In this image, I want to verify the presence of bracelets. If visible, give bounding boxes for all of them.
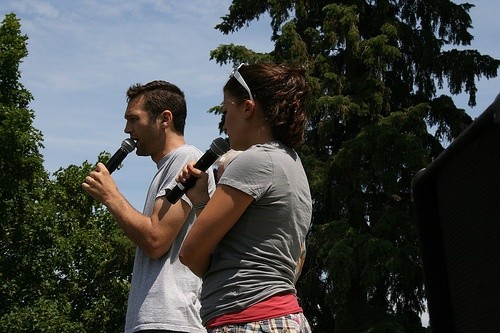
[191,199,209,210]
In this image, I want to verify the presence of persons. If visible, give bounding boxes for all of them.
[82,79,216,333]
[175,60,317,333]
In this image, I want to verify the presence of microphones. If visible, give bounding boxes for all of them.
[166,137,229,204]
[105,138,135,175]
